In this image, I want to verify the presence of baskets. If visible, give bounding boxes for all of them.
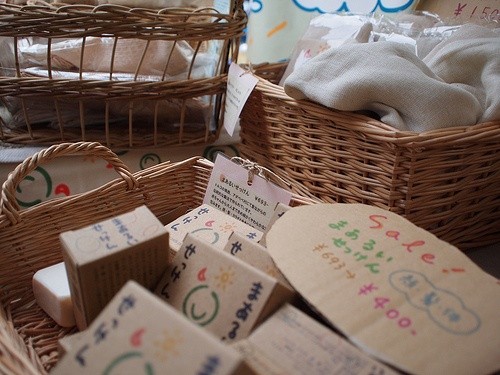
[0,141,324,375]
[239,60,500,251]
[0,0,248,149]
